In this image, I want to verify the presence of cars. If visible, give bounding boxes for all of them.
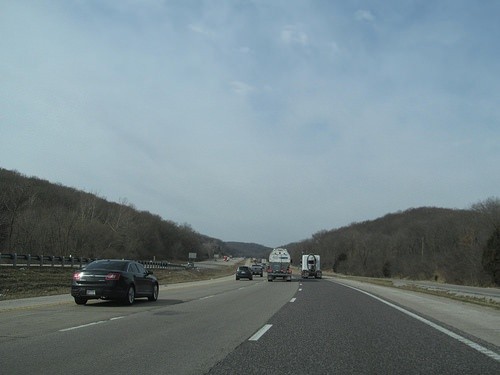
[187,260,194,267]
[251,264,264,277]
[235,265,253,281]
[71,260,159,305]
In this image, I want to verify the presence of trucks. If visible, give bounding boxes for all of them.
[301,253,323,279]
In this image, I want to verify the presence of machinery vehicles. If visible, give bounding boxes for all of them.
[266,247,293,282]
